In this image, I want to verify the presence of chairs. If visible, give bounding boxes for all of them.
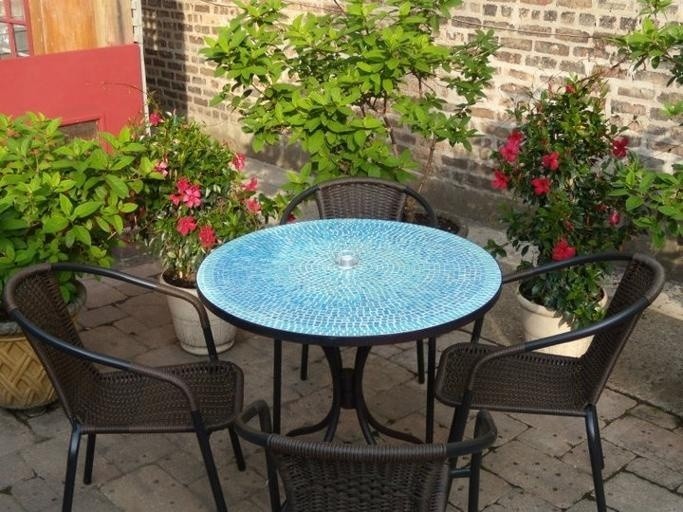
[435,251,667,512]
[235,398,497,512]
[279,178,436,384]
[4,261,244,512]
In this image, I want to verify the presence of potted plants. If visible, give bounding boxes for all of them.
[0,111,164,411]
[199,0,505,241]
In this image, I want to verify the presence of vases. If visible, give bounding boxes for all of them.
[159,272,236,356]
[514,284,608,356]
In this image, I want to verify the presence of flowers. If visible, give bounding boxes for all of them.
[480,75,642,330]
[121,112,295,282]
